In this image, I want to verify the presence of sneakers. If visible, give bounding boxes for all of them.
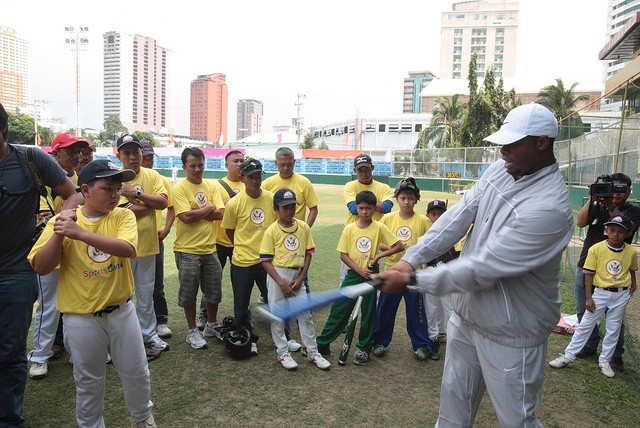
[185,326,207,349]
[307,353,331,370]
[598,361,615,378]
[29,362,48,380]
[373,340,385,356]
[301,346,331,356]
[575,350,596,357]
[437,333,448,344]
[353,348,368,366]
[202,323,223,340]
[257,296,264,304]
[148,336,170,351]
[414,347,427,360]
[272,339,302,353]
[549,352,571,369]
[277,353,298,370]
[249,342,258,355]
[69,353,111,365]
[195,321,206,330]
[609,357,624,372]
[155,324,172,338]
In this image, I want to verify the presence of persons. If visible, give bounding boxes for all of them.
[66,137,96,365]
[29,135,88,380]
[0,103,77,426]
[260,147,318,352]
[342,155,394,321]
[113,134,169,352]
[259,189,330,371]
[425,201,439,361]
[574,173,639,373]
[372,177,430,360]
[370,101,574,428]
[196,150,256,331]
[138,139,175,337]
[221,159,291,347]
[27,158,155,428]
[549,215,638,378]
[171,147,224,348]
[301,191,405,365]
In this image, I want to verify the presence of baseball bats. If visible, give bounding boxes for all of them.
[338,263,373,366]
[254,279,384,323]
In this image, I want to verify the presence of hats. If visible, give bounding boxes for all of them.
[140,141,158,158]
[74,159,137,192]
[79,137,96,152]
[482,102,559,146]
[117,134,143,153]
[603,215,631,232]
[426,200,446,214]
[273,188,300,207]
[239,157,267,177]
[50,131,89,151]
[354,154,372,171]
[392,177,421,201]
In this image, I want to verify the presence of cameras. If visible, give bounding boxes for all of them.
[589,174,628,203]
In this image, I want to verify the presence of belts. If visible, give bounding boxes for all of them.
[94,298,131,318]
[597,287,627,292]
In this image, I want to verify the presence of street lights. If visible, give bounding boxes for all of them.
[64,27,89,137]
